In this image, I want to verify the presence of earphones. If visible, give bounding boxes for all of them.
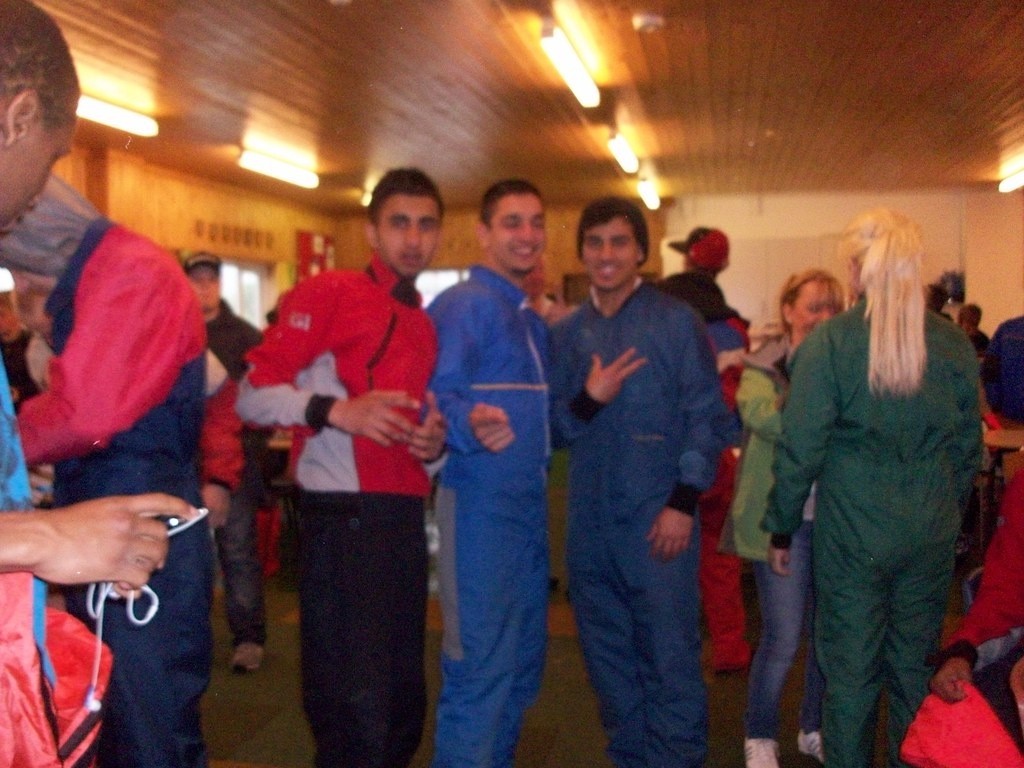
[85,685,101,713]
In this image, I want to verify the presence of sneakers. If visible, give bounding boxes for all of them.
[797,729,825,764]
[744,737,781,768]
[231,642,264,671]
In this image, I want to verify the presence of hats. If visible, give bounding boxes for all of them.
[183,251,222,273]
[670,226,729,270]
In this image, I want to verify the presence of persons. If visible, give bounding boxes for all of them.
[666,204,1023,767]
[552,197,723,767]
[236,165,450,768]
[0,216,263,768]
[421,177,550,768]
[0,0,200,768]
